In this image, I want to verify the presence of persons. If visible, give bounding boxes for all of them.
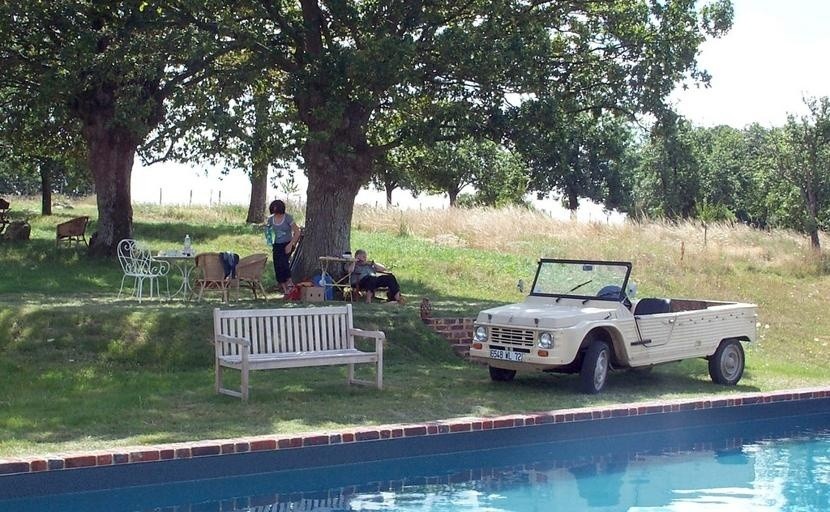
[347,249,400,304]
[265,200,300,293]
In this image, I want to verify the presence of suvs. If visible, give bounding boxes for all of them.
[490,448,758,512]
[469,257,759,391]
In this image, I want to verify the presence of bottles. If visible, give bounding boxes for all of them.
[266,230,273,246]
[184,234,191,254]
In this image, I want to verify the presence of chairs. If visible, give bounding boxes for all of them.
[634,297,673,315]
[356,260,388,301]
[117,238,170,304]
[596,286,632,310]
[188,251,239,303]
[55,217,89,249]
[228,253,269,303]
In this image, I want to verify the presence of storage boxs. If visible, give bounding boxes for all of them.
[299,287,324,304]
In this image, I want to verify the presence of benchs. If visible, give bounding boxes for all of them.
[213,303,387,404]
